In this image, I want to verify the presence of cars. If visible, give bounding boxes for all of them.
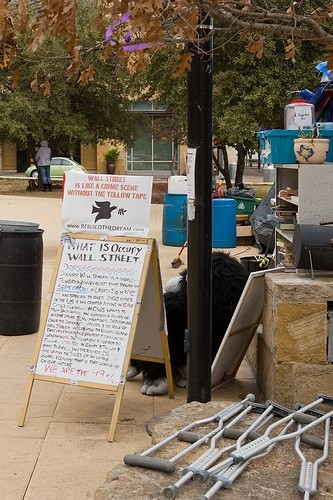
[25,156,88,184]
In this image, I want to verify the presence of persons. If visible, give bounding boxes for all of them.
[34,140,53,192]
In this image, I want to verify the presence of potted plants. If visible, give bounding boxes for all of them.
[293,122,331,163]
[102,147,120,174]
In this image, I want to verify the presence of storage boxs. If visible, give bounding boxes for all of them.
[318,127,333,163]
[258,128,315,163]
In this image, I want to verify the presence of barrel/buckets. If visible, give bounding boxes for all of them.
[0,220,44,336]
[211,197,237,248]
[263,170,275,182]
[162,193,187,247]
[230,165,237,179]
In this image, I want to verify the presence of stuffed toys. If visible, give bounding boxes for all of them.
[124,249,247,397]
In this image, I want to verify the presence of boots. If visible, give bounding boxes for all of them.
[48,184,52,191]
[41,184,49,192]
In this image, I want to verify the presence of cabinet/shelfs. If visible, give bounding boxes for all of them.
[272,159,333,274]
